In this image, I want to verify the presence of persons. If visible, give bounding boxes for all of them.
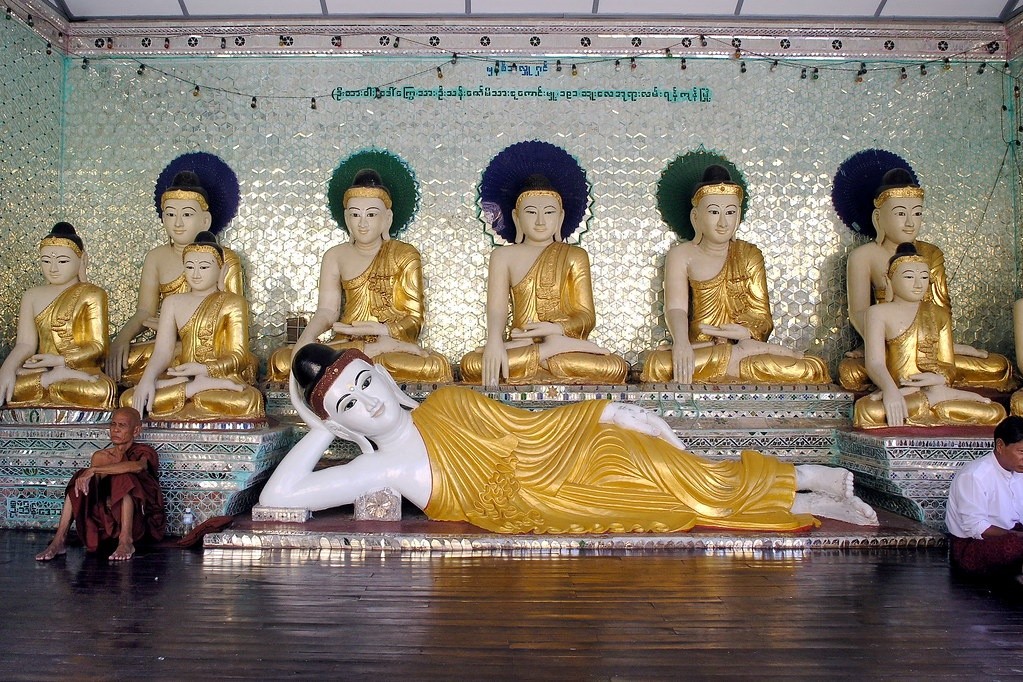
[851,242,1007,425]
[100,170,260,389]
[35,406,173,560]
[257,342,880,536]
[458,174,629,385]
[946,415,1023,604]
[268,169,453,383]
[839,167,1011,389]
[119,231,267,419]
[640,166,832,384]
[0,221,117,410]
